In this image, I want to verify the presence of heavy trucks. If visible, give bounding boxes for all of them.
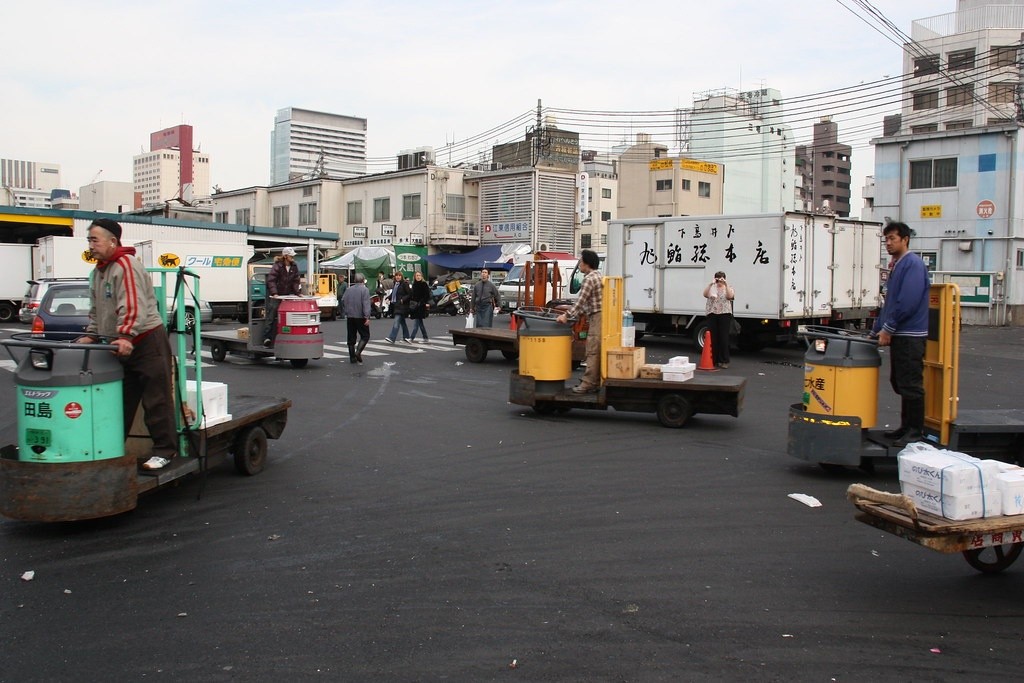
[549,210,884,355]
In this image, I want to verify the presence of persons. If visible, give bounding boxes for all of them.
[341,273,371,365]
[868,224,930,448]
[75,219,177,469]
[557,249,604,393]
[385,271,409,344]
[337,275,348,320]
[470,268,503,327]
[405,271,430,344]
[263,247,303,345]
[375,271,410,292]
[703,271,735,369]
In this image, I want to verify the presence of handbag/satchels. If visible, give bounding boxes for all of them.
[466,311,474,329]
[408,300,417,309]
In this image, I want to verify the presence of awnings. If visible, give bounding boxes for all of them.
[537,252,577,260]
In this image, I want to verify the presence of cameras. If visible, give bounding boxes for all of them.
[716,280,722,283]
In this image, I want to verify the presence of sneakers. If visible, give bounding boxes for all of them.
[143,454,171,468]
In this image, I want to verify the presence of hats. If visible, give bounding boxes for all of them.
[282,246,298,257]
[92,217,122,246]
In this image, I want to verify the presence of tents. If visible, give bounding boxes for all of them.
[318,246,396,287]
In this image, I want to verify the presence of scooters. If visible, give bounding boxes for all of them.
[380,280,475,319]
[364,279,385,319]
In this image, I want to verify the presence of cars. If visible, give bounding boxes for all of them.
[29,283,91,341]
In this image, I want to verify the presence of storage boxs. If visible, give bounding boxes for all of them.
[185,380,232,430]
[899,451,1024,520]
[237,326,249,340]
[607,314,697,382]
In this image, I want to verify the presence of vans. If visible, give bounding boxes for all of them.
[498,259,577,314]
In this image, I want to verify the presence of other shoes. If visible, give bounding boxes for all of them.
[400,339,407,344]
[893,427,922,447]
[884,426,909,439]
[406,338,413,346]
[386,337,395,344]
[419,339,431,344]
[263,338,271,346]
[572,378,596,394]
[722,361,730,369]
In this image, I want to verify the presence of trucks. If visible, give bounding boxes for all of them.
[136,239,273,324]
[0,235,141,324]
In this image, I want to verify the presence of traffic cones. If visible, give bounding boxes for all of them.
[696,330,720,371]
[509,314,518,331]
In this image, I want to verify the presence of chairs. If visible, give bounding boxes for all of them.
[57,303,76,314]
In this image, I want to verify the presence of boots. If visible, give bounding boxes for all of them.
[349,346,357,364]
[355,340,367,363]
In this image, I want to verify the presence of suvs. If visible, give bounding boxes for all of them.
[18,277,214,334]
